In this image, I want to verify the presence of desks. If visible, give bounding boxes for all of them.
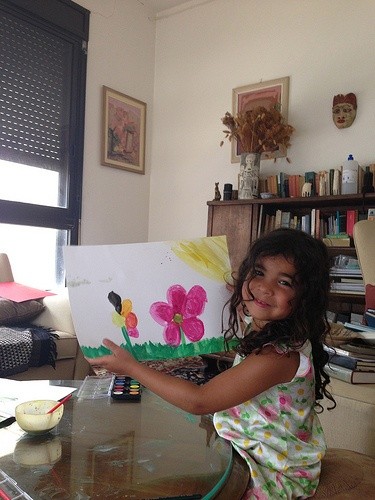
[0,377,249,500]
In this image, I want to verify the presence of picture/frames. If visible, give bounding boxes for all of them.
[232,75,290,163]
[101,84,147,174]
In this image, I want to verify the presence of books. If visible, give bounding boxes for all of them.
[264,209,358,247]
[323,344,375,385]
[259,170,341,199]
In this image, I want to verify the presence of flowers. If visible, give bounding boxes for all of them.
[220,101,296,166]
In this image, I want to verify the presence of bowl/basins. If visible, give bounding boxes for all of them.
[260,193,275,198]
[14,399,64,436]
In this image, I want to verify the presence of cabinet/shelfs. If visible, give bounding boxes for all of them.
[206,193,375,300]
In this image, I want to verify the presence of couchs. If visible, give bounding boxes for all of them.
[0,252,89,380]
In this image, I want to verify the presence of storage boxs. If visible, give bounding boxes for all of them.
[323,237,351,248]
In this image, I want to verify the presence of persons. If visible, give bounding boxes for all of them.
[83,228,336,500]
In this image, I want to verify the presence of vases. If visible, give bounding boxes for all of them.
[237,151,261,199]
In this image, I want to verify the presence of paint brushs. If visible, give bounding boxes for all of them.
[46,392,73,414]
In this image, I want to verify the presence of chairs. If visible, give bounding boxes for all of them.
[352,220,375,312]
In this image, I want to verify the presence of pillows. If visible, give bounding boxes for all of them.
[307,448,375,499]
[0,298,45,326]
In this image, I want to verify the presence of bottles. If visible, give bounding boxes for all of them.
[341,154,358,195]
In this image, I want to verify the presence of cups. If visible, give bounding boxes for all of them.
[223,184,233,200]
